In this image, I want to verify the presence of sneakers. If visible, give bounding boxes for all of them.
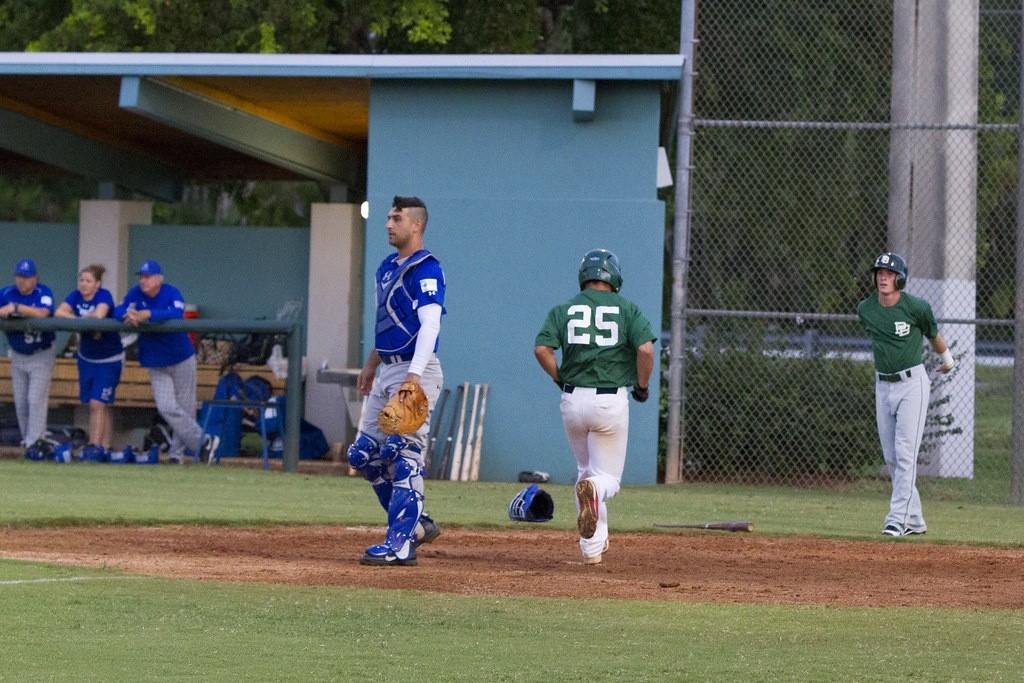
[360,543,418,566]
[903,527,927,537]
[881,523,902,537]
[168,457,184,466]
[583,538,610,564]
[575,479,599,539]
[197,434,220,465]
[411,512,441,550]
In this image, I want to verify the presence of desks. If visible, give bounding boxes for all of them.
[196,400,286,469]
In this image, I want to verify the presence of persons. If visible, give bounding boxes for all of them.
[110,260,220,465]
[346,195,447,567]
[857,254,956,536]
[533,249,658,564]
[55,263,125,463]
[0,258,56,460]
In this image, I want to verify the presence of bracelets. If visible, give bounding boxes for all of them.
[14,302,19,311]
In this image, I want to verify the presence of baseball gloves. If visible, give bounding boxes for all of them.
[377,380,429,436]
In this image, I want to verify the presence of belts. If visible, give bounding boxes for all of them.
[563,385,619,395]
[380,353,413,365]
[877,370,911,384]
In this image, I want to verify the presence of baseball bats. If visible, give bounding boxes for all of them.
[421,381,489,481]
[653,521,755,533]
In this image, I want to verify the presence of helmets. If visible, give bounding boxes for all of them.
[578,249,623,292]
[135,260,161,276]
[14,258,36,278]
[508,482,554,522]
[870,252,908,289]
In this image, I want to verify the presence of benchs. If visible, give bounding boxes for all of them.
[1,358,288,409]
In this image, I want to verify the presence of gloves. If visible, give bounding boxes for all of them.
[631,385,650,403]
[935,349,955,375]
